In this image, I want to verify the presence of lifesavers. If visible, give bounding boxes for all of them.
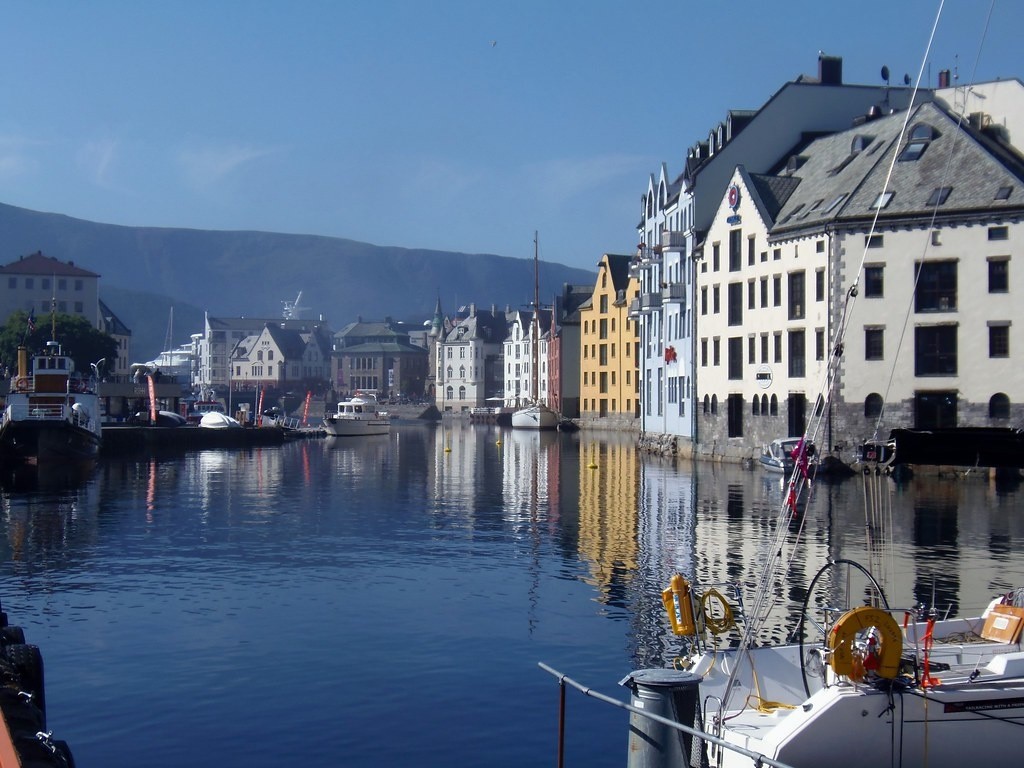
[828,605,905,680]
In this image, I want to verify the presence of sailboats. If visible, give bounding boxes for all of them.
[621,17,1024,768]
[510,229,561,430]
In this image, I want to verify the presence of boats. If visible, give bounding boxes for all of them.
[187,384,226,426]
[200,411,241,429]
[758,438,827,475]
[133,411,187,427]
[322,394,390,436]
[0,273,103,480]
